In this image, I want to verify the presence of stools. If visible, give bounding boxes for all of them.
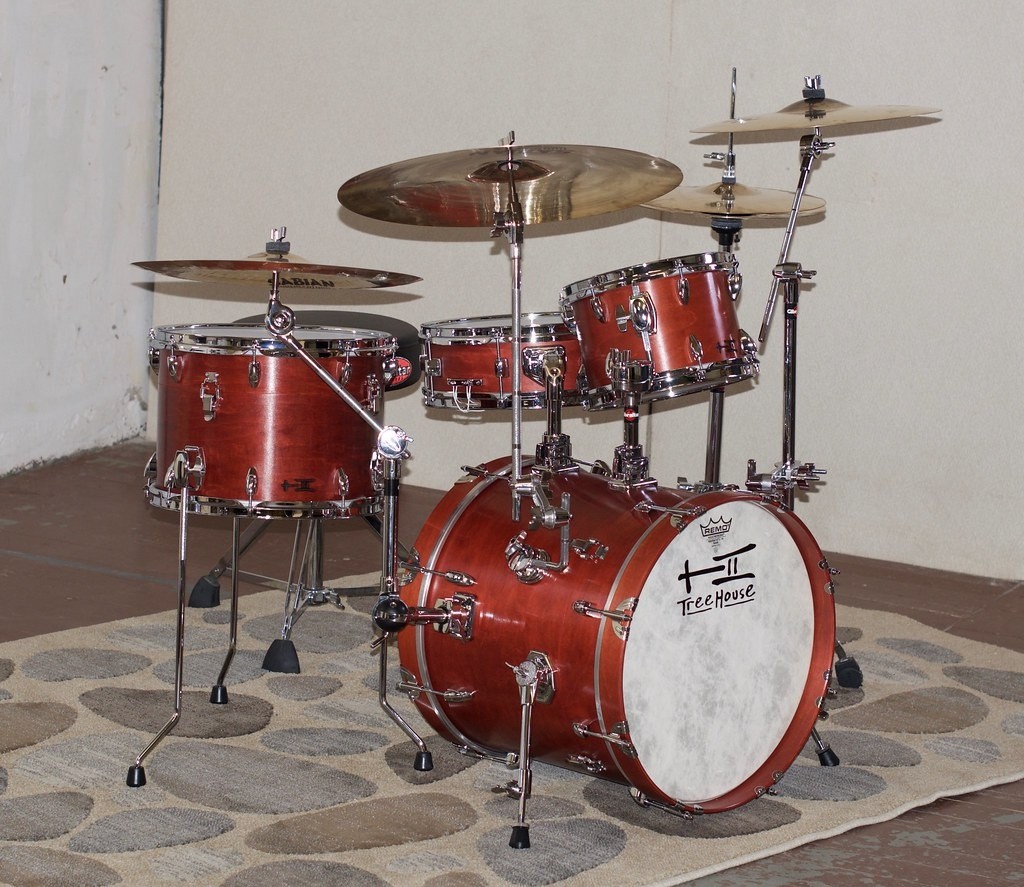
[184,308,421,679]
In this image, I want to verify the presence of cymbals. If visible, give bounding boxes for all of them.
[637,179,827,217]
[336,142,684,227]
[689,97,944,134]
[128,259,424,290]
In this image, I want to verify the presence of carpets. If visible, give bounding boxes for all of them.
[1,566,1024,886]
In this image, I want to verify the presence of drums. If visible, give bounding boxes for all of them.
[418,312,592,410]
[561,252,760,410]
[147,324,397,519]
[397,455,835,815]
